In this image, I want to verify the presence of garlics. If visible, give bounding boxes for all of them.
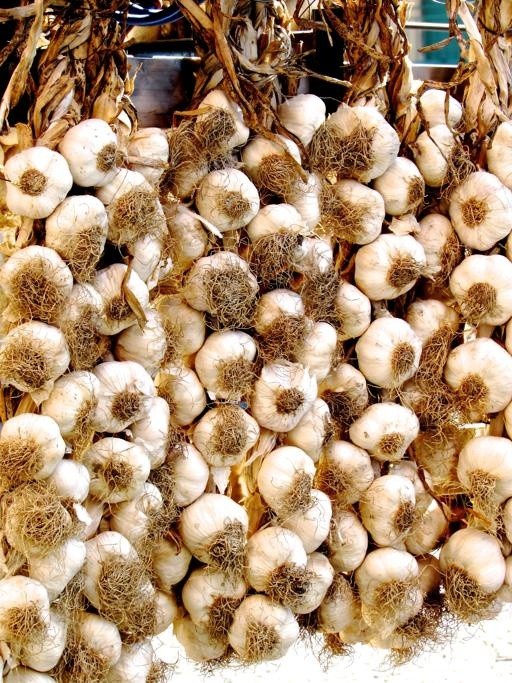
[0,87,511,681]
